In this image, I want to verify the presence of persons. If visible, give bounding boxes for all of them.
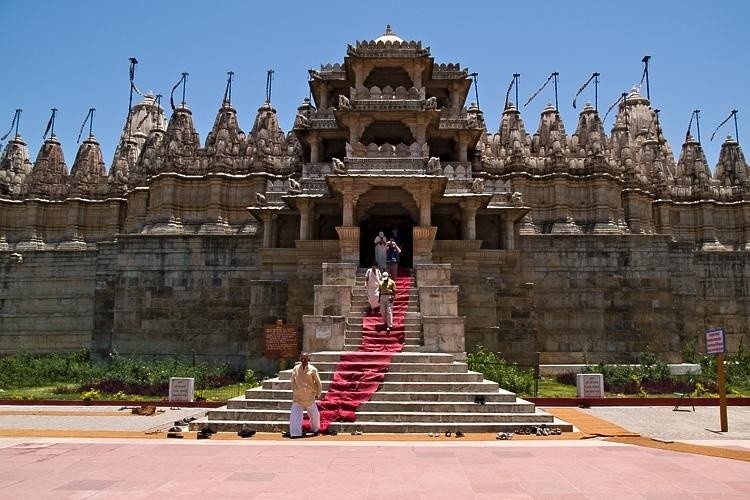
[390,224,400,242]
[377,271,397,330]
[374,231,387,273]
[384,237,402,280]
[364,262,392,315]
[288,352,323,438]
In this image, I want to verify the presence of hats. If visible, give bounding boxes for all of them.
[381,272,389,280]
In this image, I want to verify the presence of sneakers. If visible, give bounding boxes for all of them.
[428,424,561,440]
[167,417,256,439]
